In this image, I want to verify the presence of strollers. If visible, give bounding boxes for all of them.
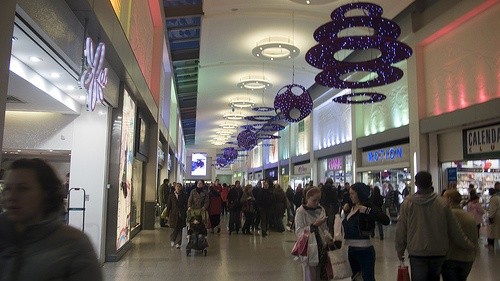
[186,207,208,257]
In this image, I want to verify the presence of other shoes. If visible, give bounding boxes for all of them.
[176,243,181,249]
[171,242,175,247]
[236,231,238,234]
[229,232,231,235]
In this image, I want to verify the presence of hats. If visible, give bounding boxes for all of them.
[415,171,432,187]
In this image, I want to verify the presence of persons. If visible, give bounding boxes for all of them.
[158,170,500,281]
[0,157,104,281]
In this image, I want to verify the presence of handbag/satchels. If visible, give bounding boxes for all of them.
[397,265,410,281]
[290,234,309,255]
[323,240,353,281]
[307,233,319,267]
